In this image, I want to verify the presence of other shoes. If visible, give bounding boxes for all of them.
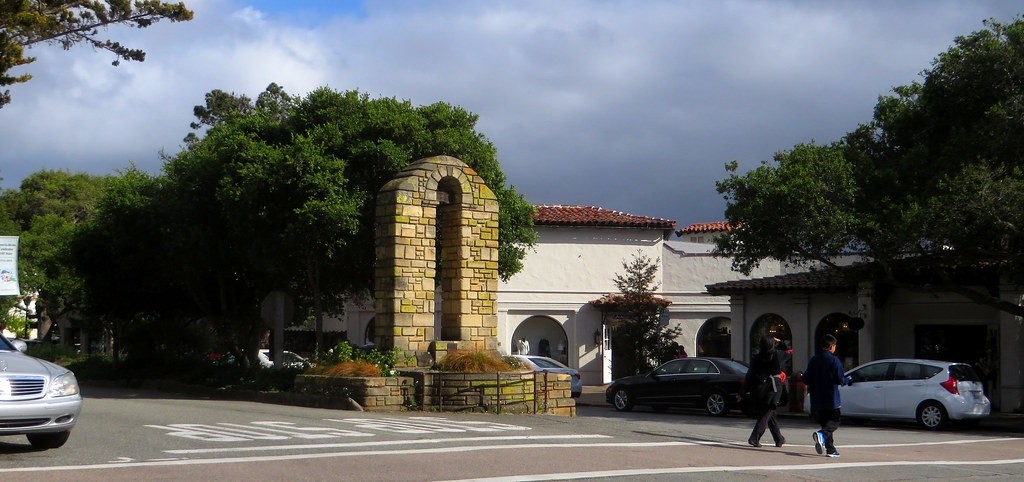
[776,437,786,447]
[749,439,761,447]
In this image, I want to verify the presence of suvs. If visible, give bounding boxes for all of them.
[803,358,993,432]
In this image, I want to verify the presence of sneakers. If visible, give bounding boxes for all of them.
[826,451,840,457]
[813,432,825,454]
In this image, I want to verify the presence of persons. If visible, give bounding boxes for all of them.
[673,346,687,359]
[518,338,530,355]
[743,333,792,447]
[802,334,851,457]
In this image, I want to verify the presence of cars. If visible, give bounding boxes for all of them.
[502,354,583,398]
[0,329,83,452]
[212,348,314,369]
[606,357,790,418]
